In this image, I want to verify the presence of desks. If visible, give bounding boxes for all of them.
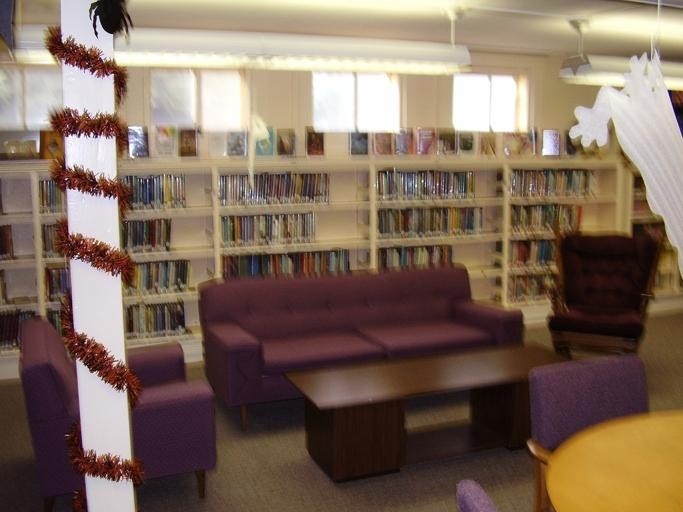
[543,404,683,512]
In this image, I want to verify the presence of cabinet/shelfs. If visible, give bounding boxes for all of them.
[0,160,72,355]
[507,155,683,320]
[120,151,505,356]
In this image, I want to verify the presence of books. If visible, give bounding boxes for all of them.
[121,217,171,254]
[220,173,329,206]
[503,130,584,158]
[509,241,557,268]
[375,168,475,200]
[510,204,582,232]
[221,212,316,247]
[507,274,556,303]
[226,128,324,158]
[222,249,350,280]
[378,244,453,271]
[123,260,191,297]
[124,301,185,340]
[511,169,590,196]
[117,174,187,211]
[349,127,496,157]
[126,125,200,158]
[377,207,483,240]
[0,131,72,350]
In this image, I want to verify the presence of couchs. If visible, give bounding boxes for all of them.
[195,264,522,429]
[544,227,660,357]
[13,312,220,499]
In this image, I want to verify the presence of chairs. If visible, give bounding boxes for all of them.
[524,352,651,512]
[456,478,498,511]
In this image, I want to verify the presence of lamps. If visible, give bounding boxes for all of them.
[560,17,683,93]
[8,0,472,79]
